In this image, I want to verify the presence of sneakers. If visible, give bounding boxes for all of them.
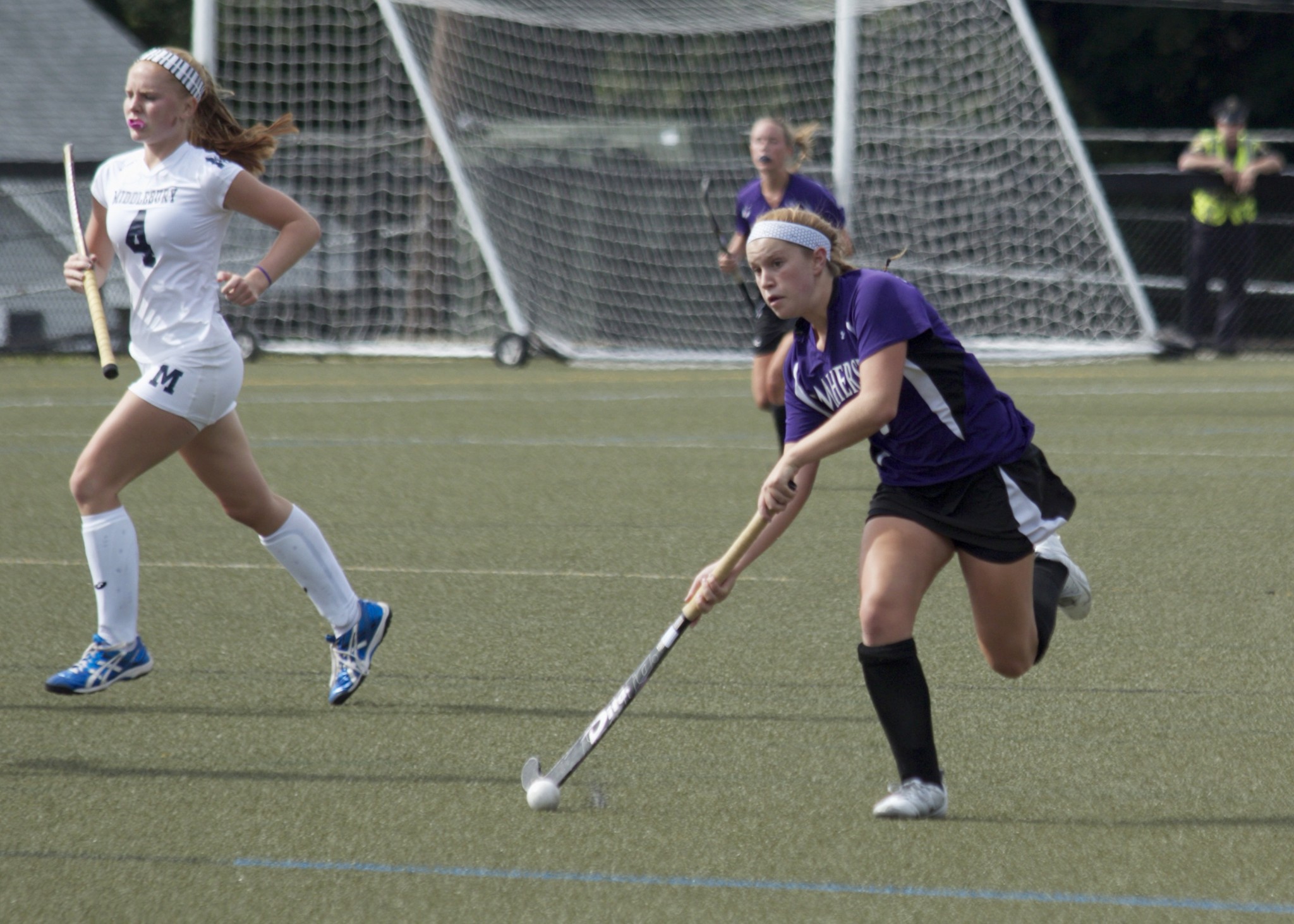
[873,779,948,818]
[326,599,393,706]
[45,634,153,696]
[1033,534,1090,621]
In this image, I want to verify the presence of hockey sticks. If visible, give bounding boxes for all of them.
[701,175,757,318]
[62,142,119,379]
[520,479,797,794]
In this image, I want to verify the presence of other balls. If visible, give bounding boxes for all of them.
[525,778,563,810]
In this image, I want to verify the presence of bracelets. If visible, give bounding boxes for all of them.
[255,266,272,286]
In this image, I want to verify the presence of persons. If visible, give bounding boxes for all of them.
[718,114,845,454]
[45,46,390,708]
[684,207,1094,815]
[1146,95,1286,359]
